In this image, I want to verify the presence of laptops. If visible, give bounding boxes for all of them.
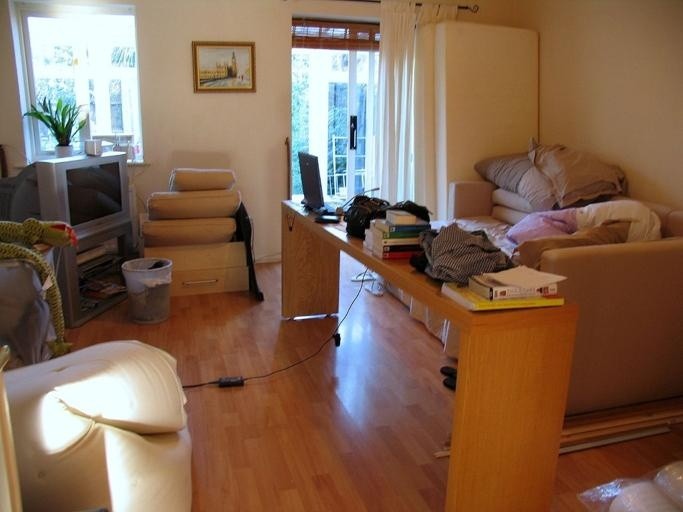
[297,150,352,215]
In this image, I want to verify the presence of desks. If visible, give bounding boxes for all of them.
[281,198,580,512]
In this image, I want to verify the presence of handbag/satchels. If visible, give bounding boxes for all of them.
[344,196,390,240]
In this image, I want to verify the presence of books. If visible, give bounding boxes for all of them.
[469,274,558,301]
[441,281,564,312]
[362,210,431,262]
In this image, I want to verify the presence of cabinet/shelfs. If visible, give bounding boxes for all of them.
[52,222,134,329]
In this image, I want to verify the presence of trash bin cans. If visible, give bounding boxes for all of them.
[121,258,172,324]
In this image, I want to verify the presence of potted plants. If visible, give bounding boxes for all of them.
[23,96,88,157]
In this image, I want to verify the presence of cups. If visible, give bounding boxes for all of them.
[84,139,102,157]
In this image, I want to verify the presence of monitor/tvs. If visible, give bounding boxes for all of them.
[33,151,133,236]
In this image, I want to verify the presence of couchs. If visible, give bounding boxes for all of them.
[448,181,683,416]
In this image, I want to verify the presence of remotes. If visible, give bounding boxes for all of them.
[316,211,342,225]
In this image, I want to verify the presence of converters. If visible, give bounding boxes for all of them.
[215,374,246,386]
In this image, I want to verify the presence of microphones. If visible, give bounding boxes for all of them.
[335,187,380,215]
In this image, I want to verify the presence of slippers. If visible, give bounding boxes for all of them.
[440,367,456,390]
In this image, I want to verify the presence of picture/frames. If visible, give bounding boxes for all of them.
[191,41,256,94]
